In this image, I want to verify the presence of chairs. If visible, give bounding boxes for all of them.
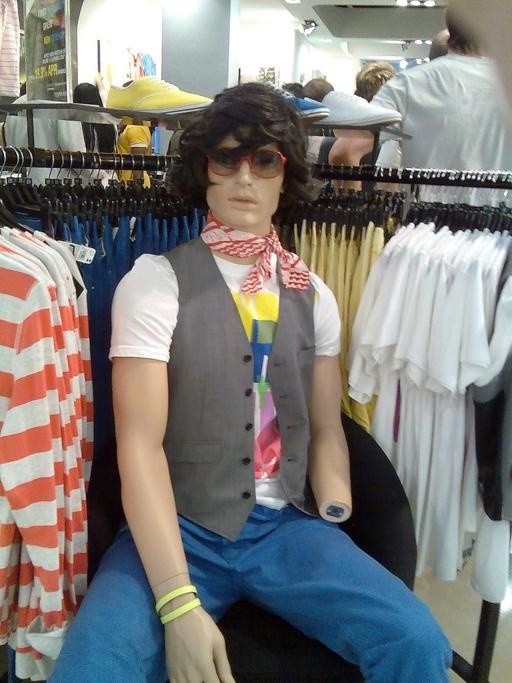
[199,406,423,681]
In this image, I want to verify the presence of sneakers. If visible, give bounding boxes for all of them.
[260,80,330,124]
[303,89,403,129]
[104,75,215,117]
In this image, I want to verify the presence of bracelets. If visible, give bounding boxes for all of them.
[153,584,203,625]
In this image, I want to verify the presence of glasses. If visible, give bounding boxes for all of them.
[205,146,288,181]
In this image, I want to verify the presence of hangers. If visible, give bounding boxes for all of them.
[0,144,512,246]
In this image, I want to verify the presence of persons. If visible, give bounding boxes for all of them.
[40,81,452,683]
[4,91,87,185]
[316,60,396,190]
[284,79,334,136]
[376,30,450,191]
[113,79,154,188]
[40,76,452,683]
[329,0,512,208]
[69,82,121,186]
